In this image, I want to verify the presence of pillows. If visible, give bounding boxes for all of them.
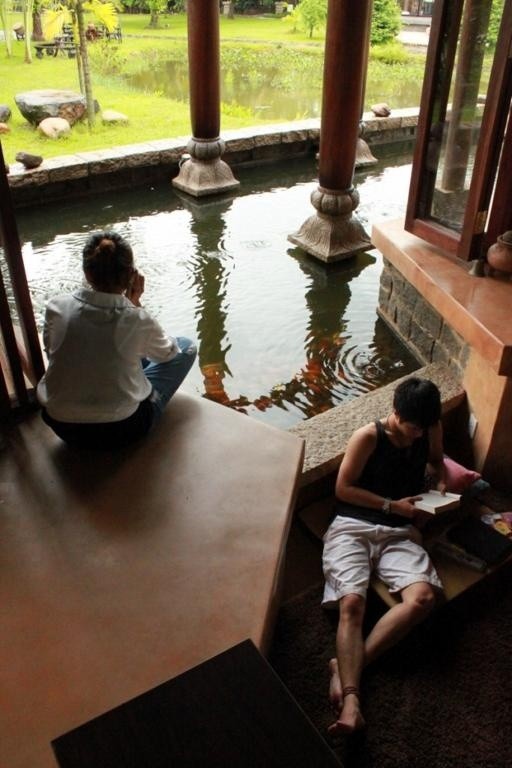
[442,454,490,503]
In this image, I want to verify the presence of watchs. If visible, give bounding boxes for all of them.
[382,498,391,515]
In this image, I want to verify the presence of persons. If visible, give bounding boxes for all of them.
[319,374,450,734]
[35,232,200,453]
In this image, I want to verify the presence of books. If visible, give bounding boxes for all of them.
[413,487,464,517]
